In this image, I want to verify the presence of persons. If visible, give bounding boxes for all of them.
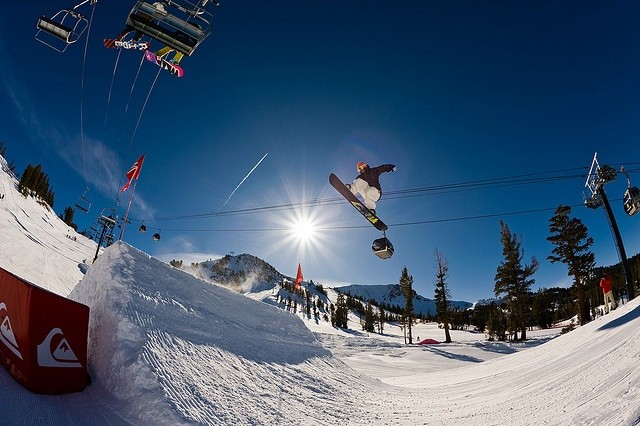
[346,161,397,215]
[599,270,617,314]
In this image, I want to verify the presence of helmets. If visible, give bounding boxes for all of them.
[357,162,367,171]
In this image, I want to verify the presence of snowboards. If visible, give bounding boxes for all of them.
[144,51,185,79]
[329,173,389,231]
[103,38,151,51]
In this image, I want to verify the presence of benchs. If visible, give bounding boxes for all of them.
[37,9,89,54]
[126,0,215,57]
[74,197,92,214]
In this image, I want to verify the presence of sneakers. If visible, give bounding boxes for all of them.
[345,184,351,190]
[370,209,376,215]
